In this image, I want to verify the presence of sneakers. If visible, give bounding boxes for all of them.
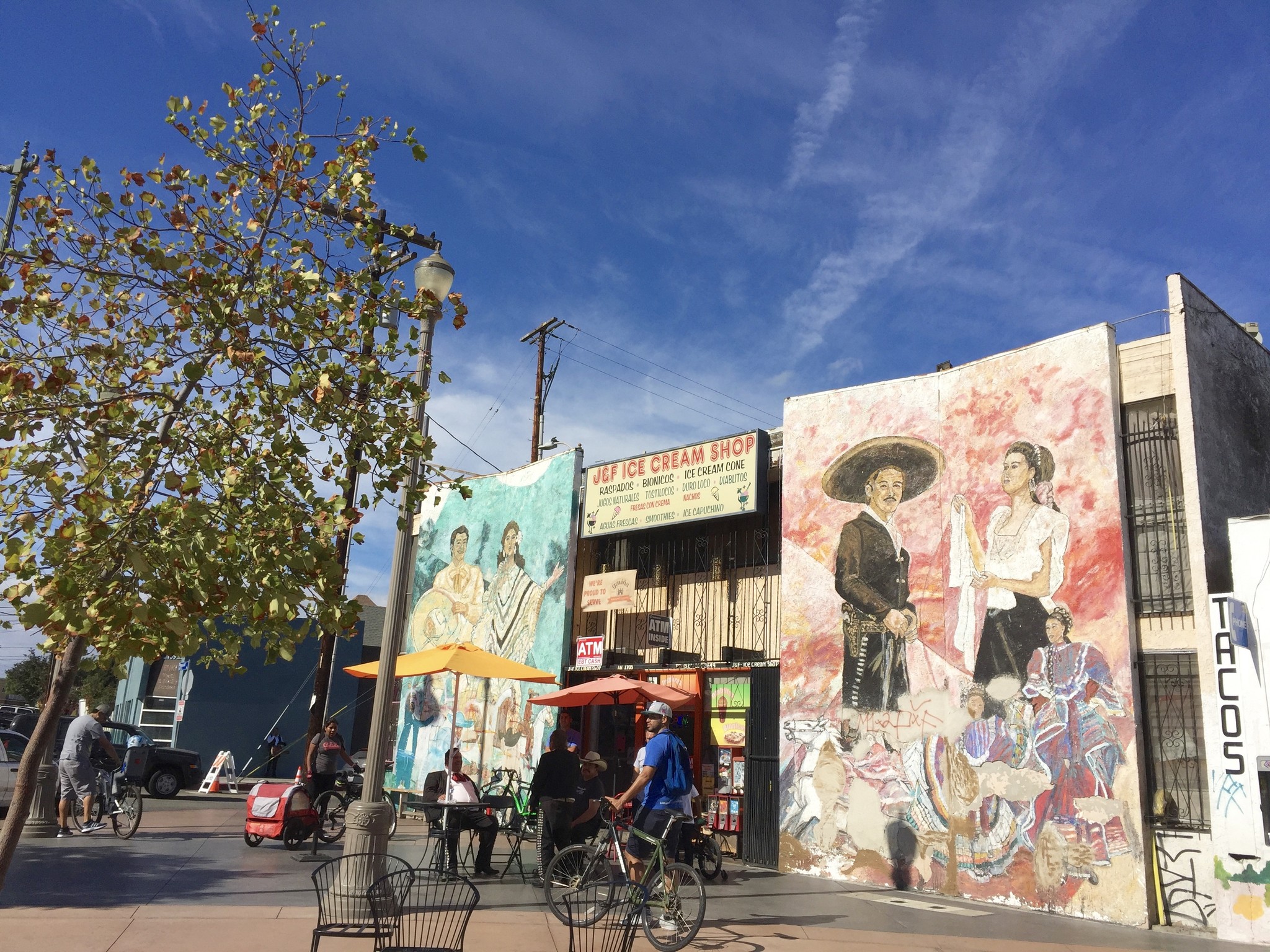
[322,830,331,838]
[649,914,678,931]
[620,909,652,927]
[81,820,108,833]
[532,878,573,888]
[56,827,73,838]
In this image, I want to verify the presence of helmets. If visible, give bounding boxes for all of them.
[127,735,147,749]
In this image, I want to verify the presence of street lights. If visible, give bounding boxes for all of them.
[322,221,457,936]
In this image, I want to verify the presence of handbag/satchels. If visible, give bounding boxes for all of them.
[271,746,281,757]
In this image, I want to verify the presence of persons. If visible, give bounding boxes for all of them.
[568,751,609,865]
[544,710,581,755]
[107,734,147,814]
[304,716,363,819]
[527,729,582,885]
[607,702,694,929]
[263,728,287,778]
[632,725,657,823]
[675,785,704,876]
[56,703,123,839]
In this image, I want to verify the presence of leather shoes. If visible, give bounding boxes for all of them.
[473,864,499,875]
[445,868,458,880]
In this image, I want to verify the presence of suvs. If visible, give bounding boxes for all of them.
[334,748,369,788]
[1,705,36,732]
[10,713,205,801]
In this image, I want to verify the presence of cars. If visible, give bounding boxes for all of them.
[0,730,62,819]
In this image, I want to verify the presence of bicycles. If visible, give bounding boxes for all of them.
[670,812,729,882]
[63,745,149,841]
[542,796,706,952]
[309,760,398,846]
[479,764,543,839]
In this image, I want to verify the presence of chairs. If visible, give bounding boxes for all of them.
[311,852,416,952]
[366,868,481,952]
[111,746,150,796]
[416,821,477,877]
[500,803,540,885]
[561,880,649,952]
[463,795,523,869]
[582,809,605,881]
[605,792,635,863]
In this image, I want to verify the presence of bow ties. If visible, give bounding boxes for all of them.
[452,773,467,782]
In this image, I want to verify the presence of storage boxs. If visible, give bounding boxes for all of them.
[706,746,745,832]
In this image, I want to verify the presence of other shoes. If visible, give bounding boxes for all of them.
[263,774,279,779]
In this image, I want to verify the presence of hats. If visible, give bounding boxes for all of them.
[640,702,673,718]
[96,704,113,723]
[577,751,607,772]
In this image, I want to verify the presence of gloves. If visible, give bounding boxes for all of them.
[306,770,313,782]
[352,763,362,774]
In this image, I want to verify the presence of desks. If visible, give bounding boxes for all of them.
[382,786,424,819]
[402,801,491,884]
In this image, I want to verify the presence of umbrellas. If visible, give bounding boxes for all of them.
[526,674,701,854]
[340,639,563,883]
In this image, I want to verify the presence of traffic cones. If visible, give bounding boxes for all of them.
[208,769,223,793]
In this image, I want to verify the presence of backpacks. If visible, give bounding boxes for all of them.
[649,731,693,796]
